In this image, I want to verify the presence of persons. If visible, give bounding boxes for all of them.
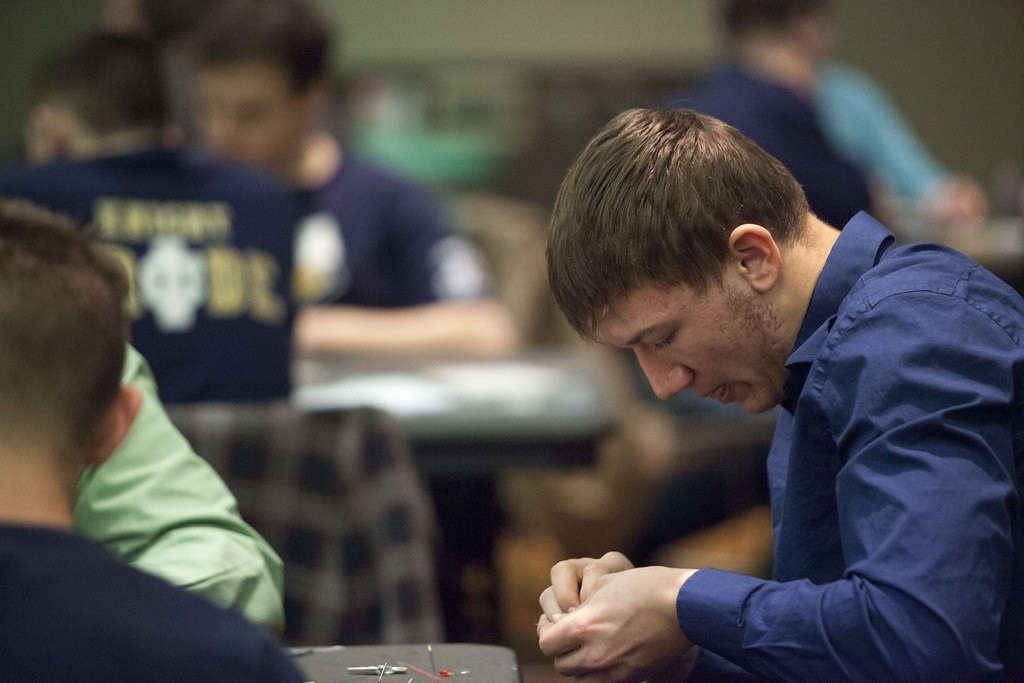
[628,1,874,423]
[0,1,519,651]
[816,56,990,230]
[536,106,1024,683]
[0,31,315,414]
[0,197,310,683]
